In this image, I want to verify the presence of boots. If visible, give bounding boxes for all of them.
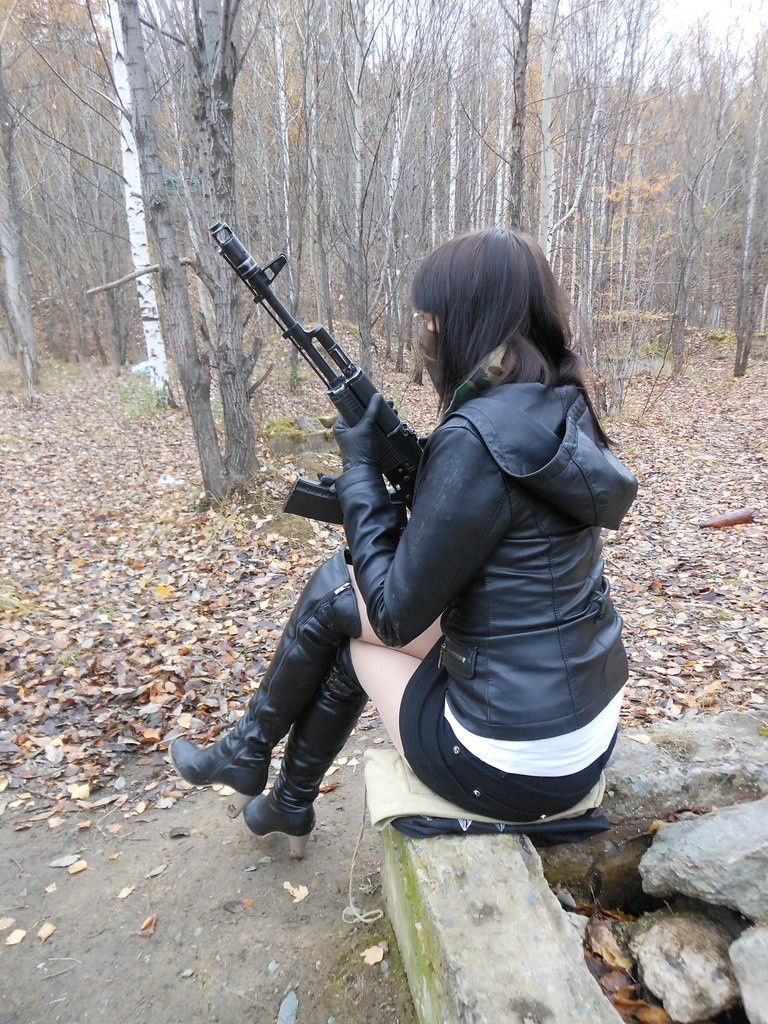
[242,635,368,859]
[168,548,362,819]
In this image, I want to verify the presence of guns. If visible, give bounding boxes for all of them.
[208,220,423,525]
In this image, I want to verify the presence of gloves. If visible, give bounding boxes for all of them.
[318,472,339,493]
[332,393,398,472]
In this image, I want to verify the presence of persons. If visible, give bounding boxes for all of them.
[168,227,639,860]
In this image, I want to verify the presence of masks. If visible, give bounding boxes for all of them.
[418,325,463,404]
